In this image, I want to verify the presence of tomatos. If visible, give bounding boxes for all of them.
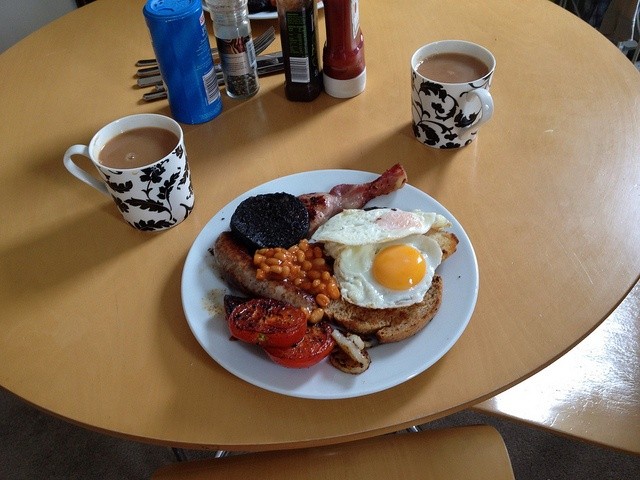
[229,300,311,347]
[261,328,335,368]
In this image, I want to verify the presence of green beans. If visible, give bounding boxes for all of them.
[251,240,341,307]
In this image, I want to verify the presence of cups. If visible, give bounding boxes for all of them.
[63,113,195,233]
[410,39,496,149]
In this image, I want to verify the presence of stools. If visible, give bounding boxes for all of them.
[143,424,514,480]
[471,280,640,455]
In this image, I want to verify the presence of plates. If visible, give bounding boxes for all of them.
[181,168,479,400]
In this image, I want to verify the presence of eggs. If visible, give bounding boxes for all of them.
[311,209,442,244]
[337,234,443,308]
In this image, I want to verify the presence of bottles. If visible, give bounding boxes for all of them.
[142,0,224,125]
[273,0,325,102]
[322,0,367,99]
[205,0,262,99]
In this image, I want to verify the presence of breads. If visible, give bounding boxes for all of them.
[326,214,460,342]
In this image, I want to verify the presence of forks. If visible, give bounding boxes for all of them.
[136,26,276,77]
